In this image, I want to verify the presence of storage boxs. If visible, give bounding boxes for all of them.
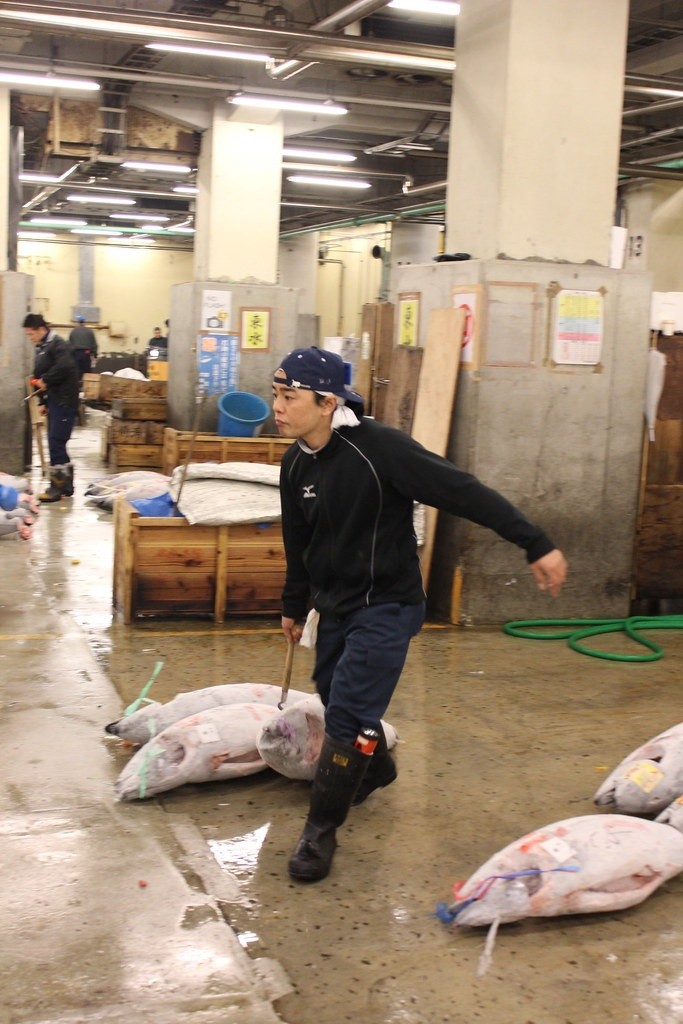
[83,374,297,626]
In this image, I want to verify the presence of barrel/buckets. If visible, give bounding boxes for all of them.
[218,391,270,437]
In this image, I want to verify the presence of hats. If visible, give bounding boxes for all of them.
[23,314,51,328]
[274,347,364,403]
[75,316,85,322]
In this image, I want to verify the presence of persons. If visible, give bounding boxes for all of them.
[69,316,98,380]
[271,346,569,883]
[22,314,82,503]
[149,327,168,347]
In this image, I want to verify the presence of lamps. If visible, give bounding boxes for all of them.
[226,91,349,116]
[0,70,101,90]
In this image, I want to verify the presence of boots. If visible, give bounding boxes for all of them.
[320,690,397,805]
[38,462,63,502]
[289,732,373,879]
[62,461,74,497]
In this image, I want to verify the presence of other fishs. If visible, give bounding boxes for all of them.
[0,472,39,539]
[257,693,399,781]
[652,796,683,831]
[439,815,683,926]
[592,722,683,813]
[84,470,176,510]
[118,702,281,801]
[104,682,313,745]
